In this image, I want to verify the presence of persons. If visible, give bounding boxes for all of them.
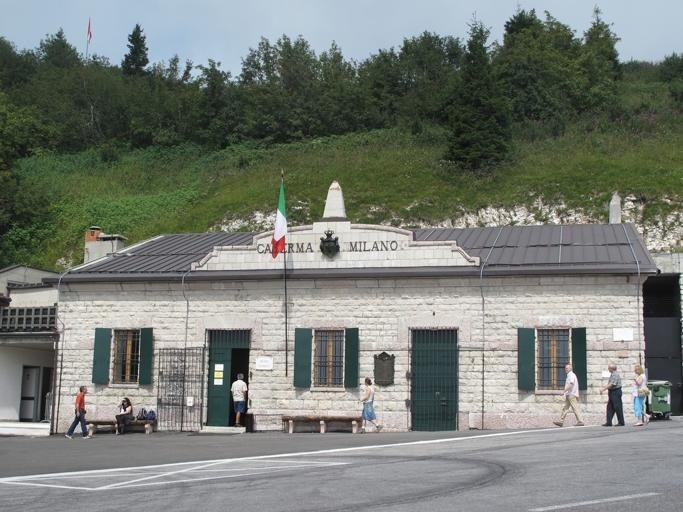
[599,363,625,426]
[114,397,132,435]
[64,385,91,440]
[230,373,248,427]
[630,364,652,426]
[356,377,383,435]
[552,364,585,427]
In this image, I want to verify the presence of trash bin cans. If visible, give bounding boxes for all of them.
[645,380,673,420]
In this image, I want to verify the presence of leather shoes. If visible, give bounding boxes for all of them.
[553,421,563,427]
[574,423,585,426]
[602,423,612,426]
[615,423,625,426]
[633,422,643,426]
[646,415,651,424]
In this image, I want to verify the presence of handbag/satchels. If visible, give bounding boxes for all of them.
[637,385,650,398]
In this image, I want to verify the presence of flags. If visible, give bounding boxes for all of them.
[270,179,289,260]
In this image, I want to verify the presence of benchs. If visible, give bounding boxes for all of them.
[85,419,156,435]
[282,415,362,433]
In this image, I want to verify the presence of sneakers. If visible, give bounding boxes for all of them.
[376,425,383,434]
[83,436,91,440]
[358,428,367,434]
[65,435,72,440]
